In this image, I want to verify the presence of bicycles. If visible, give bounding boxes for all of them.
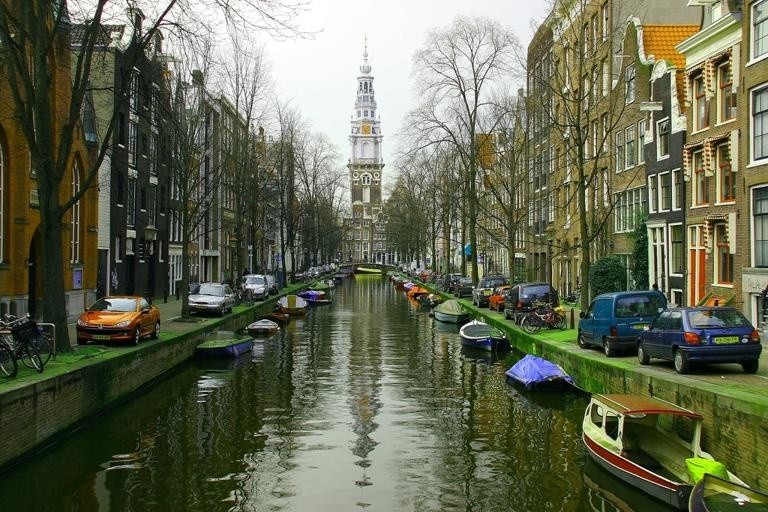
[224,280,254,307]
[288,271,320,285]
[0,312,53,378]
[519,300,568,334]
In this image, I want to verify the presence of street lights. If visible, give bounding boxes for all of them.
[302,248,309,274]
[337,252,341,265]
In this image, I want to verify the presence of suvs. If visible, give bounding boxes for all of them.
[472,275,511,308]
[635,307,762,375]
[504,281,560,324]
[242,274,268,302]
[577,290,667,356]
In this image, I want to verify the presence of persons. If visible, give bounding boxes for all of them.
[653,283,667,305]
[243,268,250,276]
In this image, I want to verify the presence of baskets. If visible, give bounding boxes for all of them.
[12,321,41,341]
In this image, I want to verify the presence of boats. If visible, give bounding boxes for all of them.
[388,273,441,305]
[357,267,381,274]
[581,392,749,511]
[197,330,255,358]
[582,456,673,511]
[430,299,470,324]
[504,353,577,392]
[247,319,279,334]
[340,269,355,280]
[258,281,335,327]
[433,318,469,334]
[333,273,347,283]
[459,344,511,365]
[460,318,506,355]
[689,473,768,512]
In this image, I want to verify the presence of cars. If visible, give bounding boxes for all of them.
[188,280,236,319]
[264,274,279,297]
[77,294,162,347]
[308,262,337,277]
[488,284,510,311]
[402,266,472,298]
